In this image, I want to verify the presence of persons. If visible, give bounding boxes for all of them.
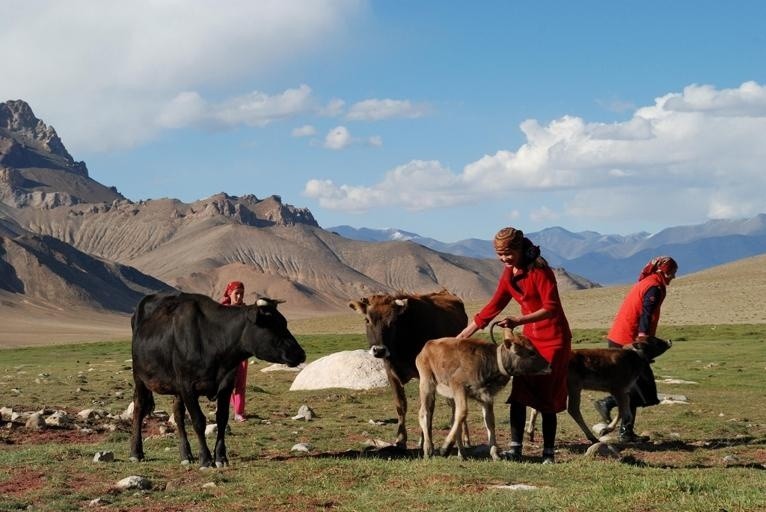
[454,227,572,464]
[217,281,250,422]
[594,256,680,443]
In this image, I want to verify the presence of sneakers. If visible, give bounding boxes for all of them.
[501,448,522,460]
[542,453,554,464]
[236,415,248,421]
[595,400,612,424]
[620,433,650,442]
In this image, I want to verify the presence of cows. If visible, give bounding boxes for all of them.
[414,331,554,461]
[526,335,673,445]
[127,290,306,471]
[347,286,471,460]
